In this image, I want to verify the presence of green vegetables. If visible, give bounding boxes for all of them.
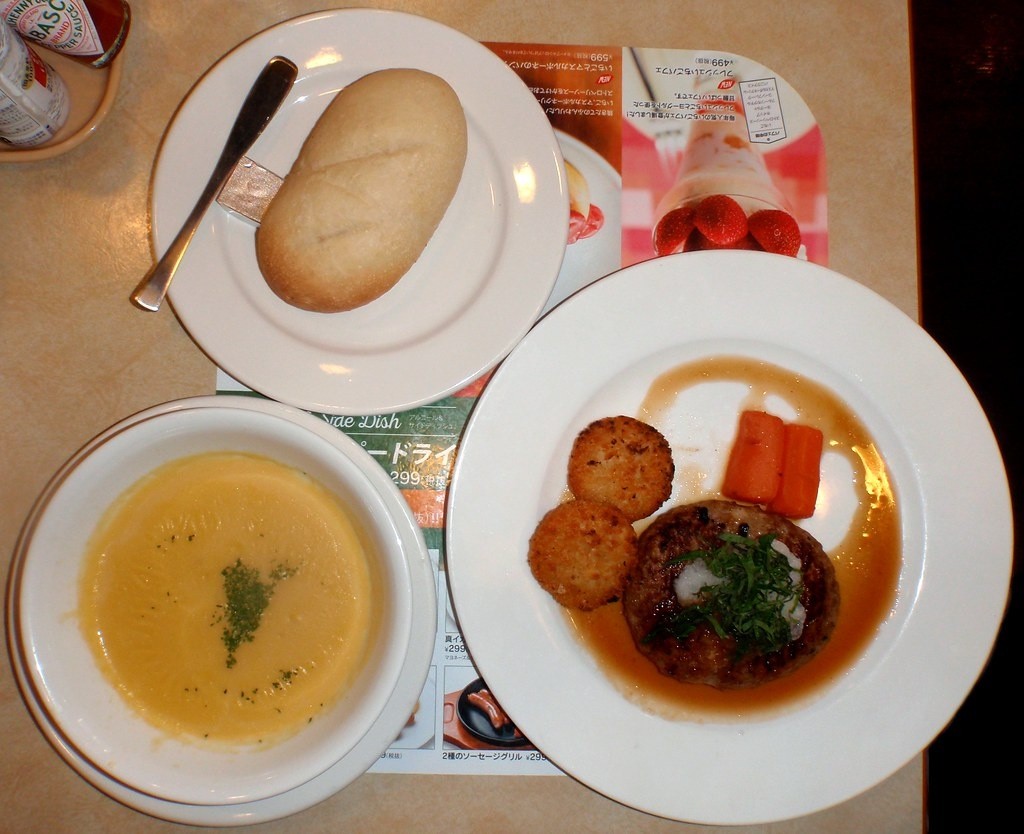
[643,540,799,657]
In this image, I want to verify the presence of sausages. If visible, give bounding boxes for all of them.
[467,690,523,738]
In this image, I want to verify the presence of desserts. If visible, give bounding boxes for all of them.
[653,85,801,262]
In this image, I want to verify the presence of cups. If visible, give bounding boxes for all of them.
[650,66,791,256]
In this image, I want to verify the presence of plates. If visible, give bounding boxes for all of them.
[443,249,1015,826]
[457,677,528,743]
[146,6,570,417]
[5,394,437,826]
[536,128,622,325]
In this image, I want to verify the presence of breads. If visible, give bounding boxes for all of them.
[256,70,467,310]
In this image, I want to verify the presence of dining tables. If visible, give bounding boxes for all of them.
[1,1,927,834]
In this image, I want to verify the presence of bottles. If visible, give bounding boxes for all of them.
[0,0,131,68]
[0,14,69,146]
